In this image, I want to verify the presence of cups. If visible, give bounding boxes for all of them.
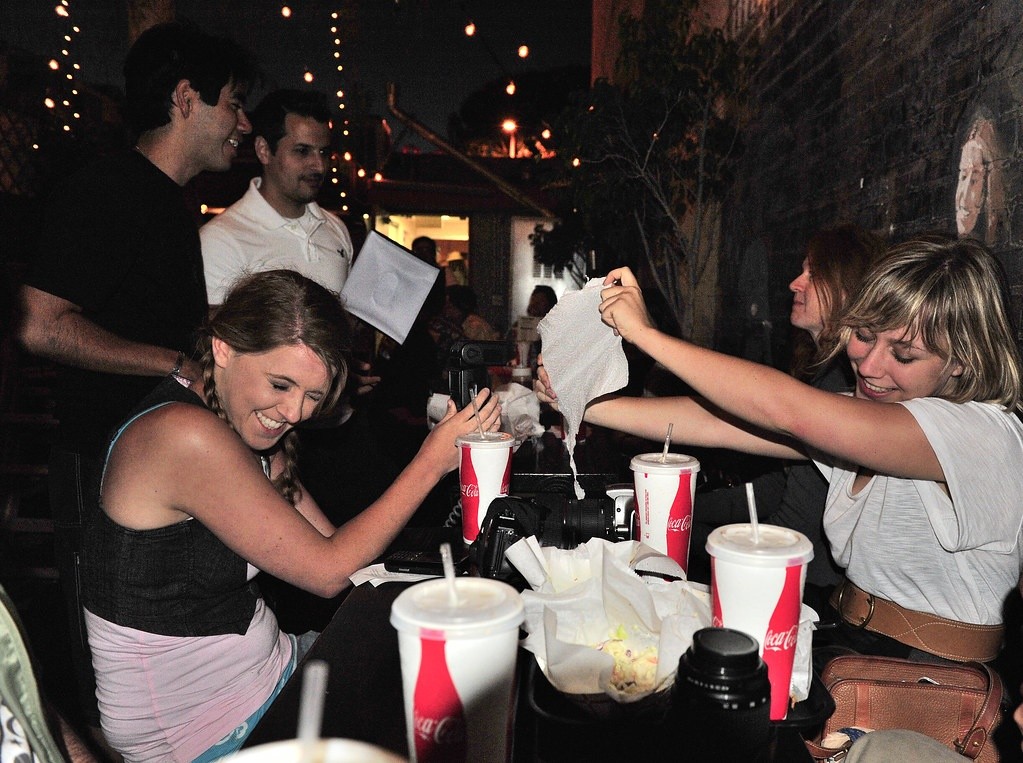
[389,577,526,763]
[216,737,405,763]
[704,522,814,721]
[453,432,516,545]
[629,452,701,583]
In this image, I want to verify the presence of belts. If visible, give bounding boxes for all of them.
[830,578,1005,661]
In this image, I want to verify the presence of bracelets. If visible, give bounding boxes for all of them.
[168,351,185,377]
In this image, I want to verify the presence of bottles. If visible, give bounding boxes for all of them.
[658,626,771,763]
[512,367,533,391]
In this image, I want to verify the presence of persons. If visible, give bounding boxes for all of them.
[507,284,557,343]
[0,22,502,763]
[536,227,1023,763]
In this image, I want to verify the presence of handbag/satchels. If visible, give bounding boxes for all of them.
[798,654,1012,763]
[467,494,634,595]
